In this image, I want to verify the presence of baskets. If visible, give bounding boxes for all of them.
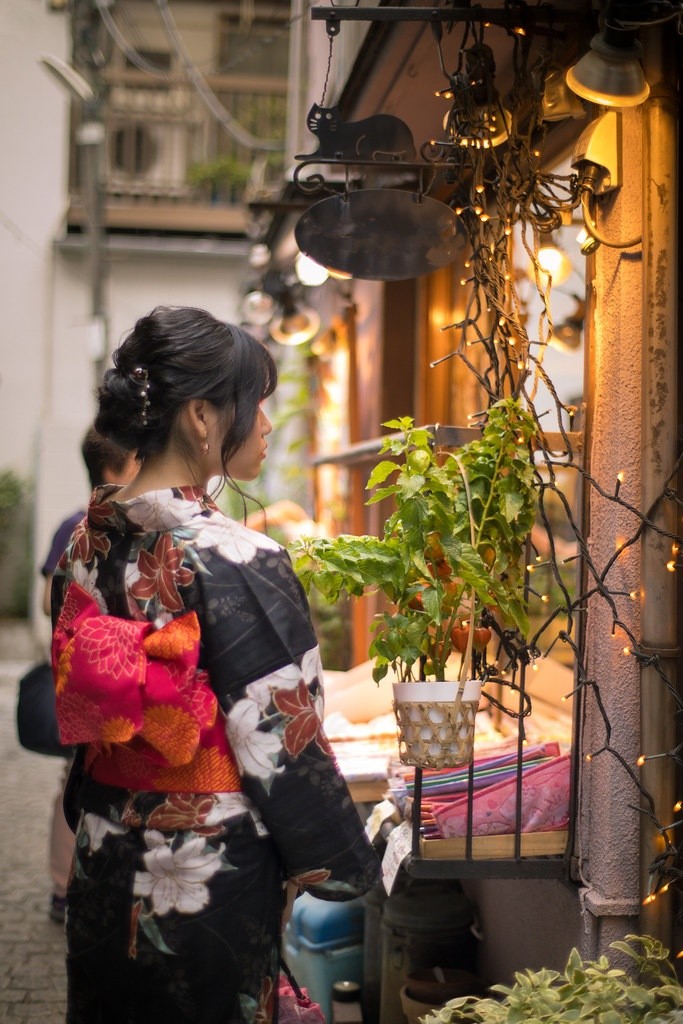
[393,701,478,770]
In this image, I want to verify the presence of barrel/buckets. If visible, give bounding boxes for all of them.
[282,880,366,1024]
[365,873,479,1024]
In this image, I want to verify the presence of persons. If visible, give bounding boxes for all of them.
[51,306,384,1022]
[40,425,142,928]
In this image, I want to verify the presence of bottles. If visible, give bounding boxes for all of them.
[332,981,363,1024]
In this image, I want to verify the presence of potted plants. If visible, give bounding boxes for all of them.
[286,395,536,771]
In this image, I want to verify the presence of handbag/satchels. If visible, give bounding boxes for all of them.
[277,957,326,1024]
[18,663,76,758]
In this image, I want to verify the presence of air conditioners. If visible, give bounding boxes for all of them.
[106,115,187,190]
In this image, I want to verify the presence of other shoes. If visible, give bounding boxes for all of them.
[49,896,67,921]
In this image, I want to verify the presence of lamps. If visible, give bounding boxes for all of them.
[442,41,512,150]
[269,297,321,346]
[565,0,650,108]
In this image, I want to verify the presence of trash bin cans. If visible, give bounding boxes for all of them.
[281,880,367,1024]
